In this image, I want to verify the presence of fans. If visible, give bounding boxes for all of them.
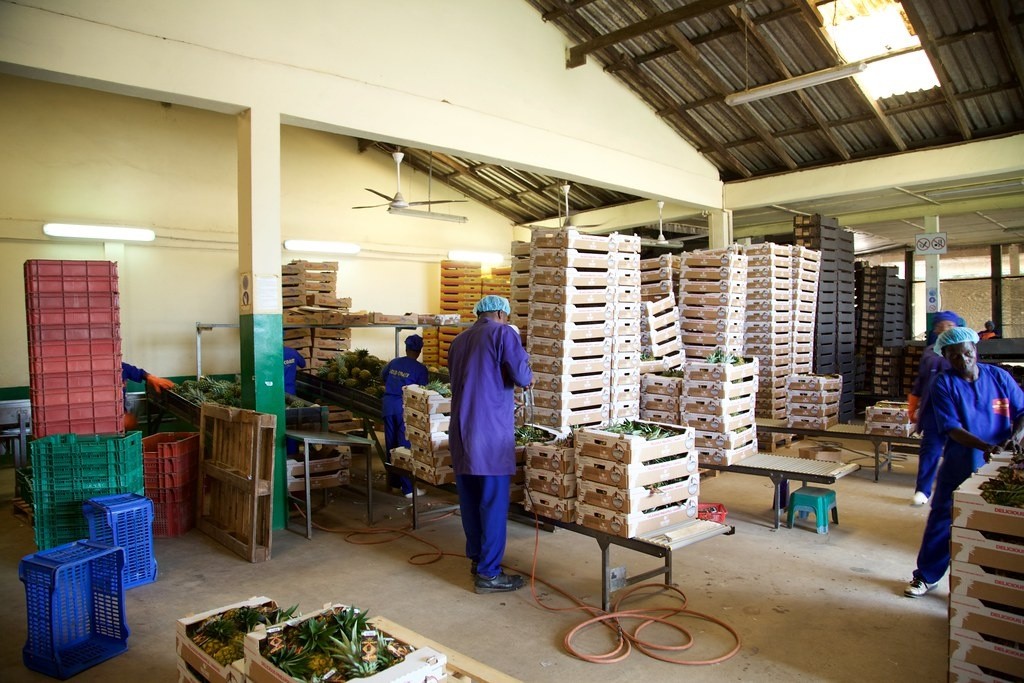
[519,181,605,236]
[352,144,470,225]
[638,200,707,249]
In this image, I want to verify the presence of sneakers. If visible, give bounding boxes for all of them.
[904,578,938,598]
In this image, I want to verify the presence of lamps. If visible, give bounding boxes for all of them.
[725,0,867,108]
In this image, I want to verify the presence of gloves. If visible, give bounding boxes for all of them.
[908,394,919,423]
[124,413,140,430]
[146,375,175,393]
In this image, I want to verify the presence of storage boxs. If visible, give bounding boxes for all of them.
[947,451,1024,683]
[19,215,924,683]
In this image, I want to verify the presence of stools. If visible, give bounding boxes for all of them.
[787,487,839,533]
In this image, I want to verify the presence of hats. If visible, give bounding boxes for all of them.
[405,335,425,348]
[932,310,959,328]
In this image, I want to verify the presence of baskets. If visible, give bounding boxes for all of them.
[696,503,728,523]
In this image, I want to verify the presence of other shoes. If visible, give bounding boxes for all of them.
[392,486,403,493]
[910,491,928,506]
[405,487,428,499]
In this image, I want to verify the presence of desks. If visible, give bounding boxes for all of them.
[385,461,730,614]
[755,420,922,481]
[699,454,849,528]
[283,429,375,538]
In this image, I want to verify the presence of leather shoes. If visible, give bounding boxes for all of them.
[475,571,524,594]
[470,565,503,577]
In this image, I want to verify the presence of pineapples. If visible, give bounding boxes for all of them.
[171,377,305,410]
[731,356,745,367]
[190,606,411,683]
[315,348,453,399]
[513,424,577,450]
[979,465,1024,509]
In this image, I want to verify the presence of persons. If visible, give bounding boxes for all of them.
[284,347,306,454]
[449,294,536,594]
[122,362,174,429]
[978,320,999,336]
[907,311,966,507]
[380,334,429,499]
[903,327,1024,598]
